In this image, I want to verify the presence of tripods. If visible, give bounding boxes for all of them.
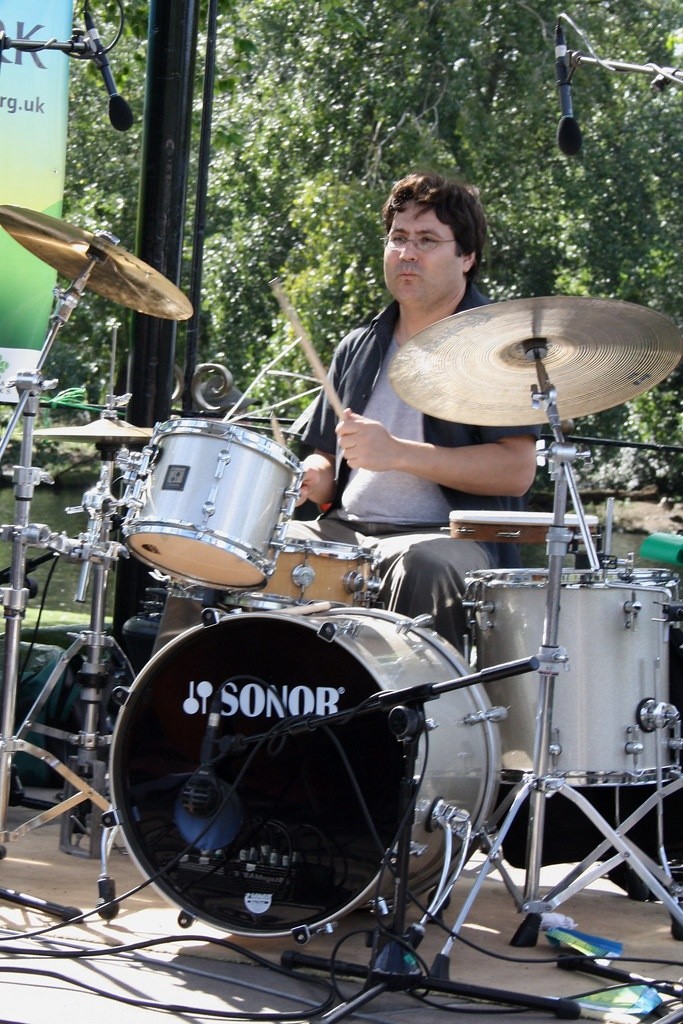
[0,256,683,1023]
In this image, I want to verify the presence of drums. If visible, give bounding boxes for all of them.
[449,509,599,543]
[121,418,304,591]
[106,608,503,937]
[211,536,375,613]
[462,568,677,788]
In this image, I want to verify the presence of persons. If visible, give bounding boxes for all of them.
[286,172,544,663]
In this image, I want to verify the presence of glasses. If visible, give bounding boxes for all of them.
[379,231,456,255]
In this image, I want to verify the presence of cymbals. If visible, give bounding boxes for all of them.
[386,295,683,427]
[17,419,154,446]
[439,510,601,545]
[0,204,193,320]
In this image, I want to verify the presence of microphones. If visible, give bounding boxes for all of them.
[556,26,582,153]
[82,12,132,130]
[180,697,222,820]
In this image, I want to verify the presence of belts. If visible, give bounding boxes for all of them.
[341,519,446,537]
